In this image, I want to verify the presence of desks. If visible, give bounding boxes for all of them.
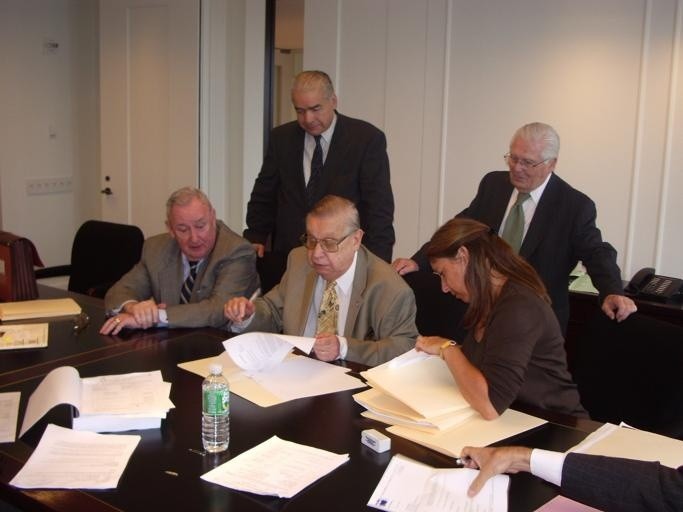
[0,283,683,512]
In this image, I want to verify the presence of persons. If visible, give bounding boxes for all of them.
[390,121,637,340]
[415,218,591,422]
[225,193,421,366]
[460,445,682,511]
[96,186,260,336]
[242,70,394,281]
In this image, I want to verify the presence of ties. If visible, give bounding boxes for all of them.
[306,135,322,209]
[501,192,530,256]
[314,281,338,339]
[179,261,198,304]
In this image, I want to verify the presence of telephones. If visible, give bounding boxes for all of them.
[623,268,683,304]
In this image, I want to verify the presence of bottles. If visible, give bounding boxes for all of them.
[201,364,231,452]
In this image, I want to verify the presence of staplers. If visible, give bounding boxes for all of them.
[73,312,90,333]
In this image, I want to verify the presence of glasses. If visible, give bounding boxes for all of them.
[504,152,548,168]
[300,232,352,252]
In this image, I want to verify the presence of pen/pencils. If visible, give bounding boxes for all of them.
[456,456,471,464]
[246,288,261,308]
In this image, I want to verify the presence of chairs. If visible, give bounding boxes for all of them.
[401,272,470,346]
[69,220,144,300]
[569,309,683,441]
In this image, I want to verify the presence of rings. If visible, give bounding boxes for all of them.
[113,317,120,323]
[321,350,325,355]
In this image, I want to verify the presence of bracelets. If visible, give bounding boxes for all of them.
[439,341,457,358]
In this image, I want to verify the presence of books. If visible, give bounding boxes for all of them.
[17,366,174,431]
[564,423,682,470]
[0,323,50,352]
[565,269,601,297]
[351,345,550,460]
[0,297,82,321]
